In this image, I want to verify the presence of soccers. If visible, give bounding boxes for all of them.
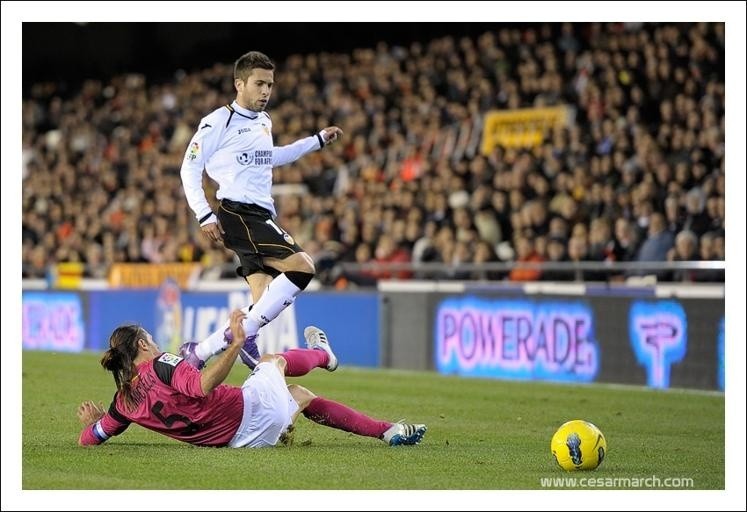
[551,419,607,474]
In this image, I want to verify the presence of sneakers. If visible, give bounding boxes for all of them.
[178,341,206,373]
[380,417,427,445]
[304,326,338,372]
[223,326,260,371]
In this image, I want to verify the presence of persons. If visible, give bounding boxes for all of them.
[21,23,724,372]
[76,310,428,450]
